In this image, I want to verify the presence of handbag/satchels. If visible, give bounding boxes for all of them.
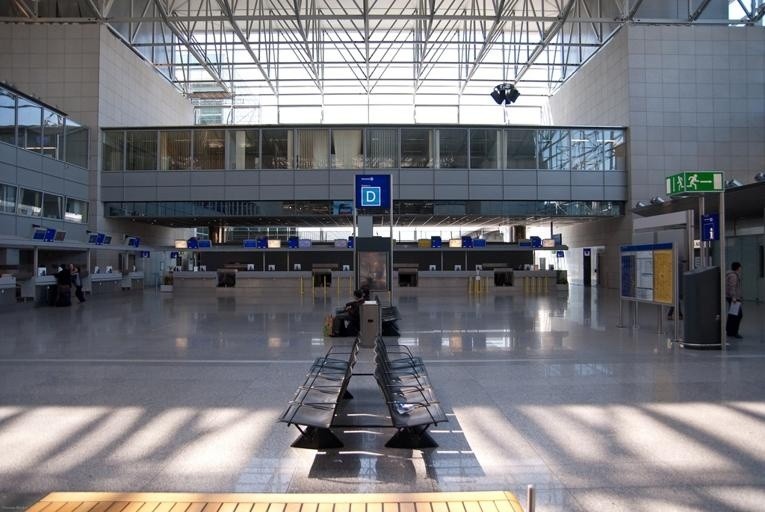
[323,314,333,337]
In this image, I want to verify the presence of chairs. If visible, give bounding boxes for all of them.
[329,287,403,337]
[371,334,449,451]
[274,332,361,452]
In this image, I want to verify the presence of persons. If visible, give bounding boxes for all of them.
[69,263,86,303]
[359,286,370,301]
[726,260,744,338]
[330,289,364,336]
[54,263,71,306]
